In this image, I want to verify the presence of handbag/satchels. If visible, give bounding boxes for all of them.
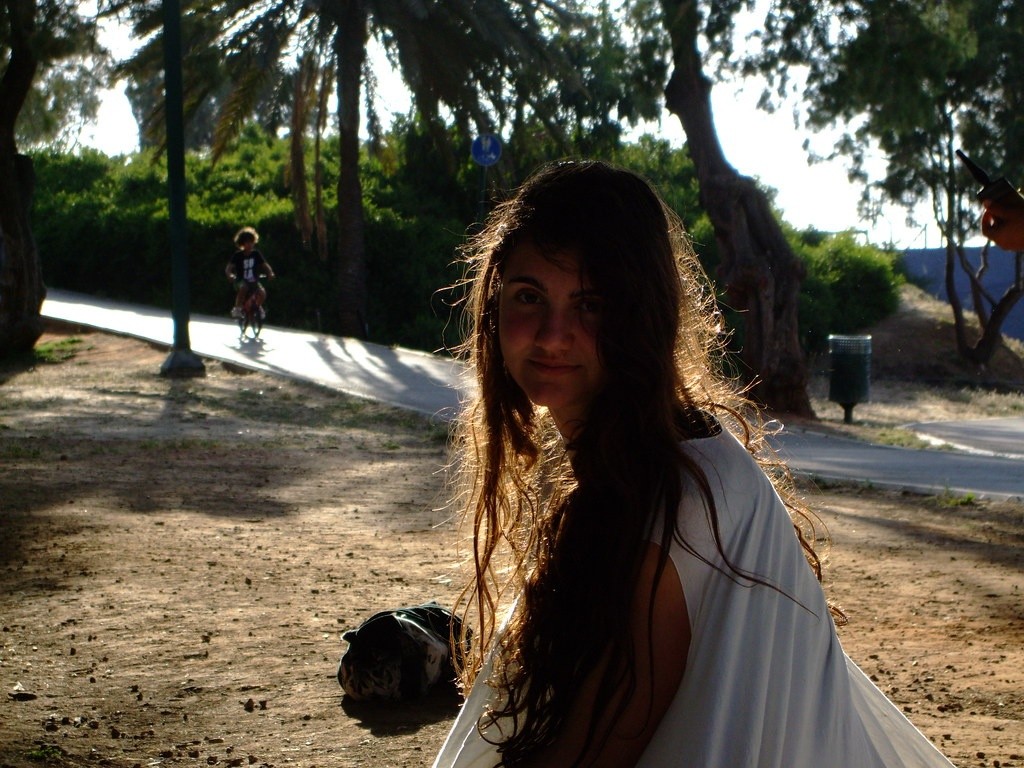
[337,600,473,705]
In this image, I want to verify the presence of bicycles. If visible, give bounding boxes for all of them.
[237,290,265,339]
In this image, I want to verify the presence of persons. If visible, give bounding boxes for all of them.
[225,228,273,319]
[982,197,1024,251]
[433,158,960,768]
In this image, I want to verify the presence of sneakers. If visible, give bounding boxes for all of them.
[231,306,242,319]
[258,306,266,320]
